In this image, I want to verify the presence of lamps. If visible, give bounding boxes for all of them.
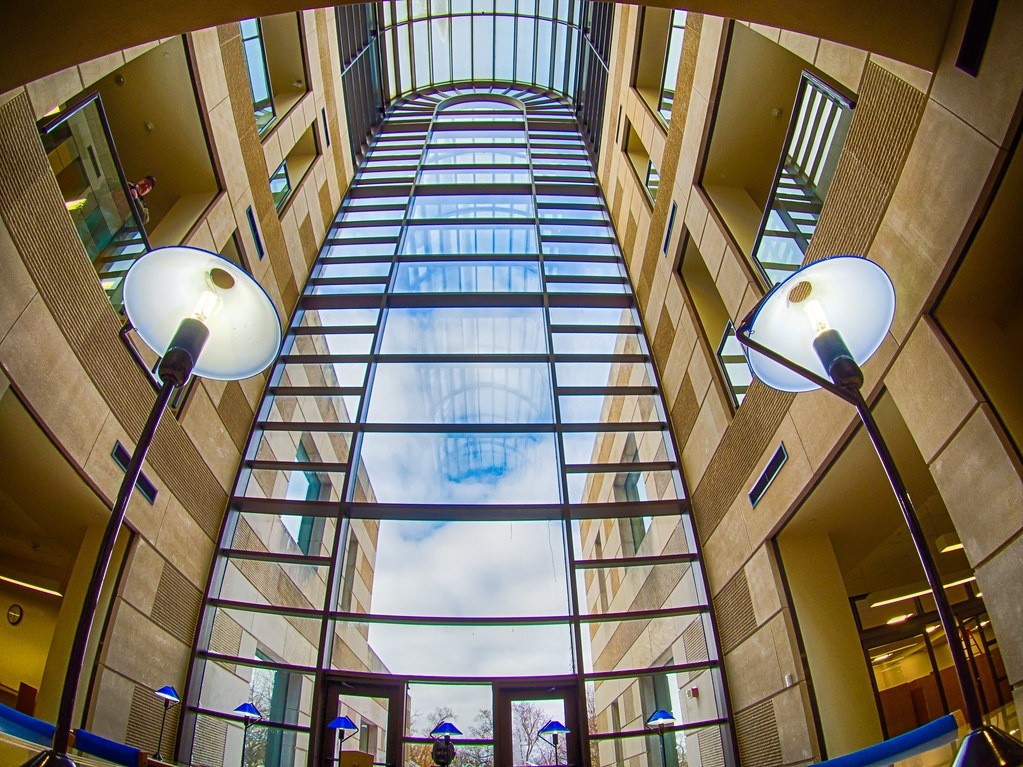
[645,710,676,767]
[537,720,571,767]
[430,721,463,767]
[152,686,180,761]
[20,247,283,767]
[328,715,359,767]
[736,255,1023,767]
[233,703,262,767]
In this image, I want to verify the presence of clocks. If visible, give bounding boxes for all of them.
[7,604,23,625]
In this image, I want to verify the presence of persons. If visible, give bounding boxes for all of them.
[78,175,157,264]
[431,738,456,767]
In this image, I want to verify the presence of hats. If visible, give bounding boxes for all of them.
[145,174,156,189]
